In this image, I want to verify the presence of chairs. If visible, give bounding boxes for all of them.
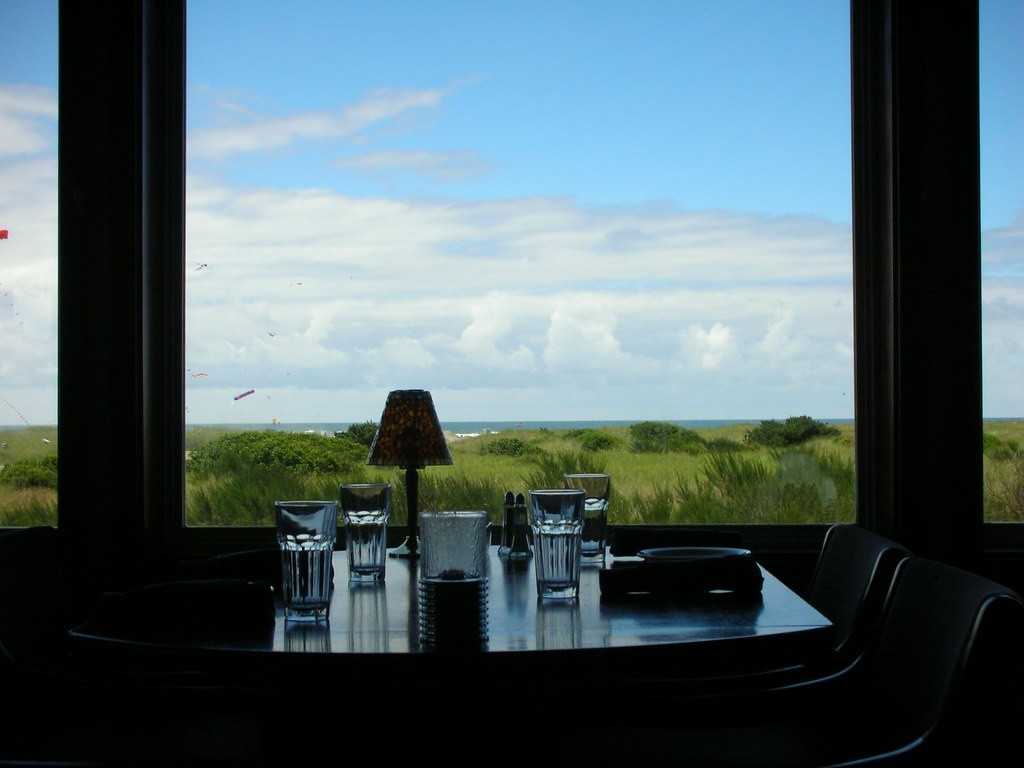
[561,523,1024,768]
[0,527,260,768]
[613,527,745,556]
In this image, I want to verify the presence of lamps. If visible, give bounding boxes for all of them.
[366,389,452,560]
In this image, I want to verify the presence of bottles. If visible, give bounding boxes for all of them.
[507,494,533,561]
[498,490,515,554]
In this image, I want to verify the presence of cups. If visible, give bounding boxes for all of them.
[528,488,587,599]
[273,499,339,622]
[563,472,611,563]
[338,483,393,585]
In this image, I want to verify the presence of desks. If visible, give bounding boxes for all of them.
[65,555,837,690]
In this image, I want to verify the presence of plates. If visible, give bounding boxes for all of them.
[637,546,753,562]
